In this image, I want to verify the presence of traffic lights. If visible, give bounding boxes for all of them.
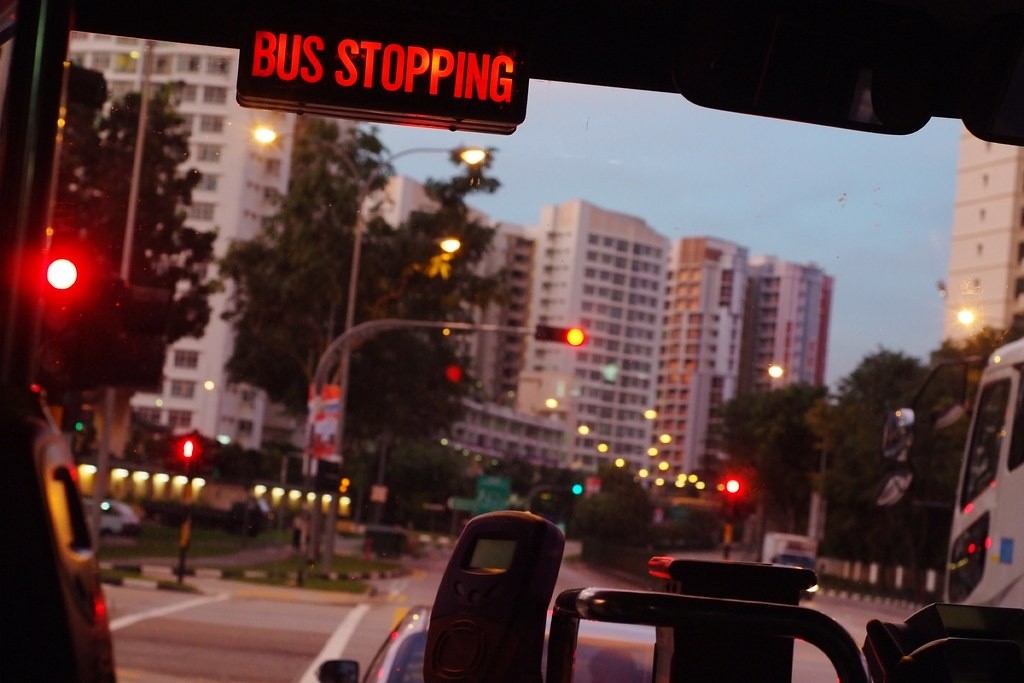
[44,252,79,292]
[725,476,740,495]
[180,436,198,461]
[442,356,464,388]
[537,325,588,345]
[572,483,584,498]
[73,419,88,434]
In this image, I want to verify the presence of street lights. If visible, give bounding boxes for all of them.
[250,124,485,575]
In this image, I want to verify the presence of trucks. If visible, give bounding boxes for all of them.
[882,337,1023,606]
[762,530,822,601]
